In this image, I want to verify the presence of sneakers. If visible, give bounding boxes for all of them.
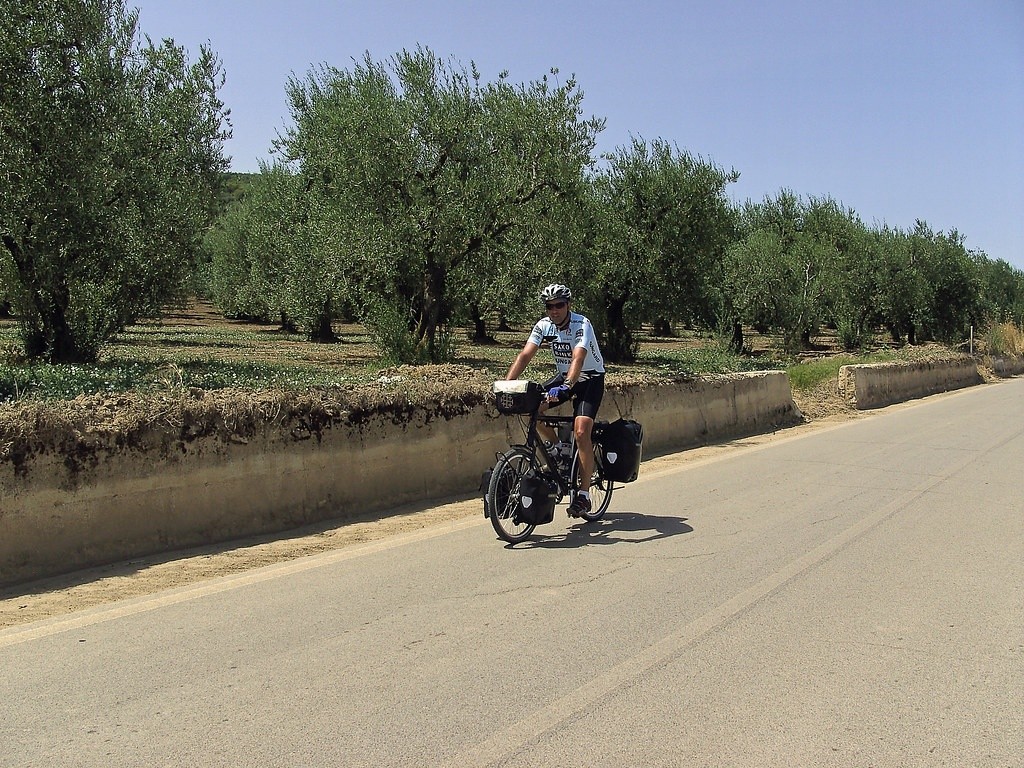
[542,453,563,470]
[566,494,591,517]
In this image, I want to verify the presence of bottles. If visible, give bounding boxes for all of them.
[545,441,564,466]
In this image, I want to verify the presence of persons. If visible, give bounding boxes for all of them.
[506,282,607,515]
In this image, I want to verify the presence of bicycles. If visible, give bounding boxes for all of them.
[484,378,644,543]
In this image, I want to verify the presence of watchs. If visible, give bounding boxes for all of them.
[564,378,573,386]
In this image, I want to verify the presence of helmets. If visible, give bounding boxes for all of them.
[540,283,571,304]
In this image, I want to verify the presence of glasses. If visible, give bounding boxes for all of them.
[546,301,568,310]
